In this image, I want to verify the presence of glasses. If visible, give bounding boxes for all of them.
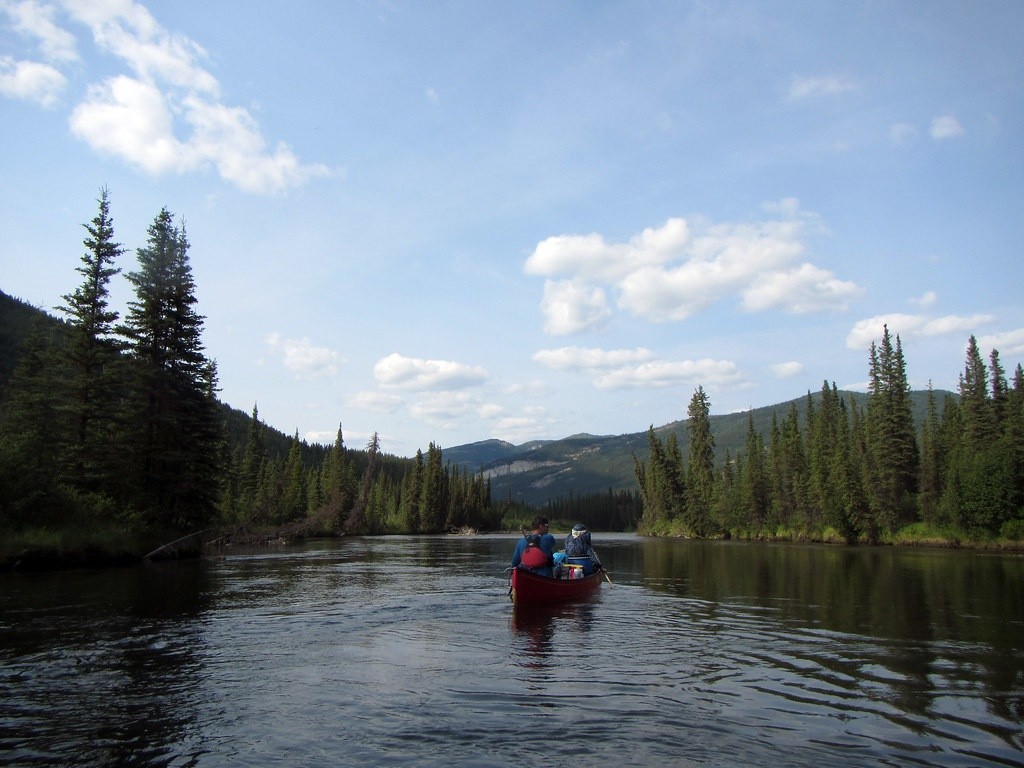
[544,523,549,528]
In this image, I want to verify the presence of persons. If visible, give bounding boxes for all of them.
[511,517,559,577]
[565,524,608,575]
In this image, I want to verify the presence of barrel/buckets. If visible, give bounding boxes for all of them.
[568,568,582,579]
[568,554,593,577]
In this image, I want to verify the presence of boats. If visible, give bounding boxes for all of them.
[505,567,603,608]
